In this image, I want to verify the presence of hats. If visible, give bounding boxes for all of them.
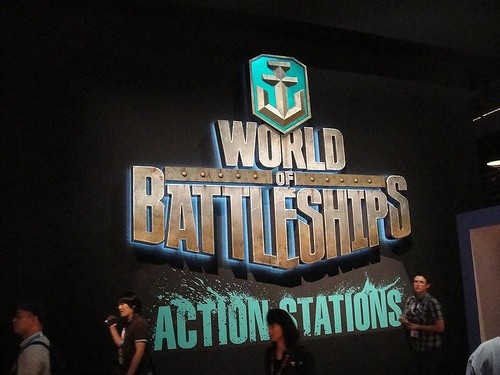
[11,295,48,322]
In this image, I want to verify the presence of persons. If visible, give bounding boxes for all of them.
[466,336,500,375]
[399,272,445,375]
[9,299,52,375]
[106,293,155,375]
[264,308,316,375]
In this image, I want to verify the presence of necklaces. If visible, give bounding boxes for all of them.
[270,347,290,375]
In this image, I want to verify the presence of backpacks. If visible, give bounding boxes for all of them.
[18,340,73,375]
